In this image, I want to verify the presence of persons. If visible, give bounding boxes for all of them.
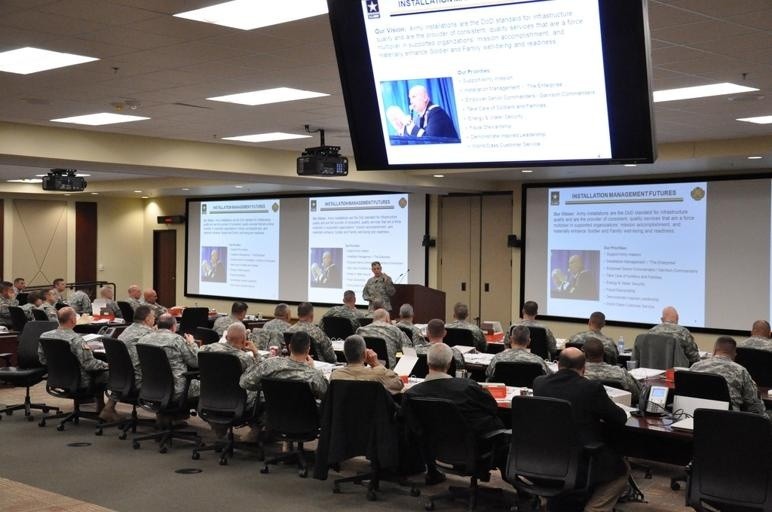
[39,307,126,424]
[533,346,631,511]
[552,255,595,299]
[580,339,640,404]
[13,278,26,296]
[116,306,157,391]
[647,305,701,369]
[50,278,67,299]
[141,289,169,315]
[503,301,557,360]
[408,343,513,485]
[569,311,617,365]
[318,290,363,334]
[285,302,337,362]
[313,252,339,288]
[329,335,404,396]
[136,313,200,400]
[239,330,326,400]
[363,262,395,312]
[739,320,772,354]
[357,307,412,370]
[0,281,17,326]
[396,84,460,144]
[94,287,125,318]
[264,303,296,355]
[410,318,465,379]
[67,287,93,316]
[126,285,143,312]
[199,321,262,391]
[692,337,769,421]
[397,304,427,348]
[443,304,486,351]
[203,249,226,283]
[21,293,43,321]
[38,288,59,324]
[212,302,248,338]
[485,327,551,383]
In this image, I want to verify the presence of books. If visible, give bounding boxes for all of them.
[82,331,103,342]
[629,367,668,381]
[453,344,474,355]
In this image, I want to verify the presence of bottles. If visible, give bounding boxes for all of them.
[617,335,624,353]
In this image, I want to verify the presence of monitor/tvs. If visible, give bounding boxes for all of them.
[325,1,658,172]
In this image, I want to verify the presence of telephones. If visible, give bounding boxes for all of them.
[630,383,668,415]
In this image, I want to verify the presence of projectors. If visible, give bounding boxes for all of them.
[42,169,86,189]
[296,144,348,177]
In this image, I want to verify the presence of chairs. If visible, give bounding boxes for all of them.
[635,335,692,371]
[117,301,134,323]
[502,395,605,512]
[565,342,583,350]
[322,317,354,342]
[259,377,341,477]
[178,307,209,337]
[16,293,29,306]
[509,326,548,362]
[395,325,413,348]
[8,306,27,331]
[31,308,49,321]
[284,332,319,361]
[132,342,205,454]
[314,380,420,502]
[602,379,624,391]
[193,327,219,345]
[734,347,772,388]
[55,303,69,310]
[0,321,63,422]
[412,354,456,379]
[400,378,506,512]
[38,338,110,431]
[492,362,543,389]
[95,338,157,440]
[686,407,772,512]
[356,318,374,327]
[192,351,264,465]
[674,370,733,411]
[442,328,473,348]
[363,336,389,370]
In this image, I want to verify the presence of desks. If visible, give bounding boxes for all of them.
[637,376,772,410]
[482,329,504,342]
[483,338,633,370]
[331,340,558,388]
[390,284,446,325]
[313,360,693,466]
[175,313,271,330]
[0,314,124,368]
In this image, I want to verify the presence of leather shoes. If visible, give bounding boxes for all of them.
[425,470,446,486]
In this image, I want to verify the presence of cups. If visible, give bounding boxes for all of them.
[410,375,417,385]
[520,389,528,396]
[627,360,636,370]
